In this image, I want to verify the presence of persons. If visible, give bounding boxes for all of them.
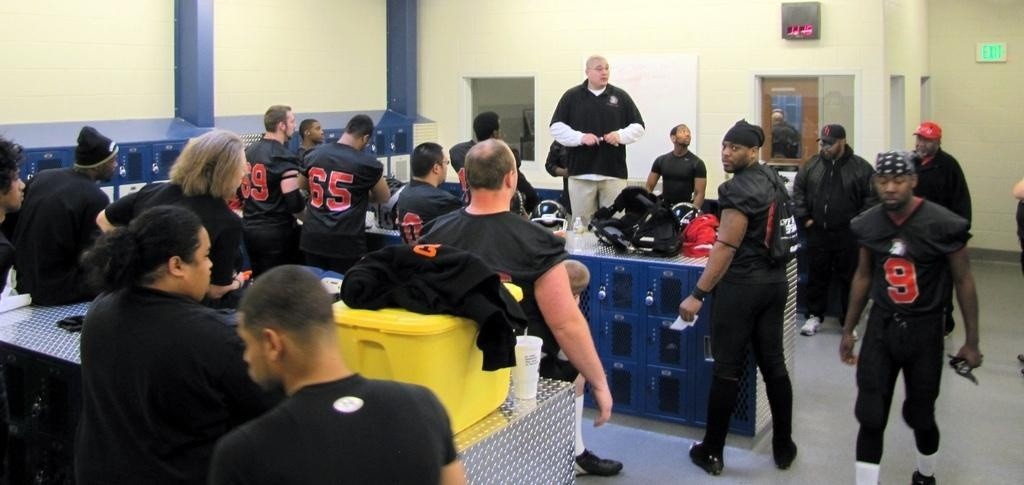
[797,121,986,341]
[394,138,625,476]
[1,105,387,302]
[78,207,463,485]
[679,118,797,476]
[1012,173,1024,375]
[545,56,644,230]
[839,148,983,485]
[643,124,707,208]
[770,108,800,159]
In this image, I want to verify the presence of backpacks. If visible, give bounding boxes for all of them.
[590,185,685,258]
[742,169,805,266]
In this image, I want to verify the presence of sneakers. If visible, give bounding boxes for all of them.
[799,315,823,336]
[842,325,861,341]
[686,441,728,477]
[575,446,625,480]
[772,440,799,471]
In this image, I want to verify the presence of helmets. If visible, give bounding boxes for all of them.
[528,199,567,234]
[670,200,706,233]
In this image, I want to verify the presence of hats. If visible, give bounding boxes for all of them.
[815,124,845,144]
[74,124,121,169]
[913,121,944,140]
[722,119,766,149]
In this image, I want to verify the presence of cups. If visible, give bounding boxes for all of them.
[513,336,542,397]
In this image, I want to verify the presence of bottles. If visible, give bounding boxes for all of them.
[573,215,584,231]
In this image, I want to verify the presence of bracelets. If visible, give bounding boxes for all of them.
[690,286,709,302]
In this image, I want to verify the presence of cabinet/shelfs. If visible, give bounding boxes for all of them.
[0,110,800,485]
